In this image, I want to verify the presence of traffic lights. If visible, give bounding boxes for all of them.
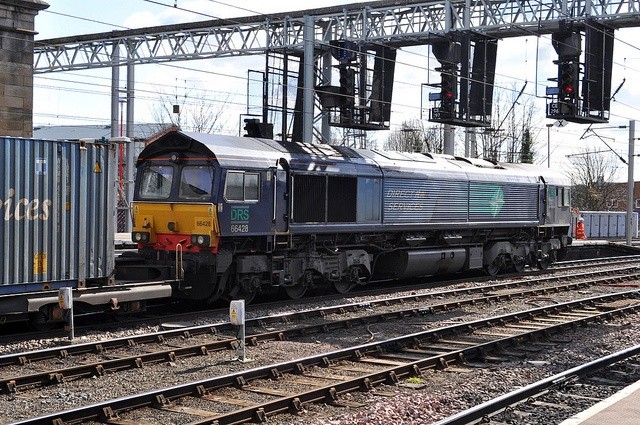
[560,62,576,103]
[439,70,453,110]
[338,67,351,106]
[241,119,262,138]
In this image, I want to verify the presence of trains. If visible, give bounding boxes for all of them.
[0,126,575,326]
